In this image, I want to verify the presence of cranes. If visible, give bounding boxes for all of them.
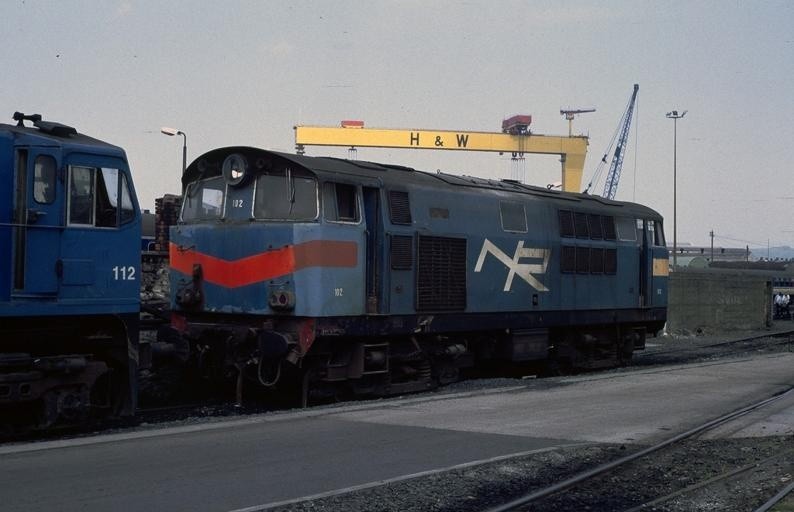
[557,106,597,137]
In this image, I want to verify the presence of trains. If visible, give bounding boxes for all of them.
[0,111,670,444]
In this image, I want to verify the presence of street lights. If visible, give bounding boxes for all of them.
[662,109,687,266]
[156,125,187,195]
